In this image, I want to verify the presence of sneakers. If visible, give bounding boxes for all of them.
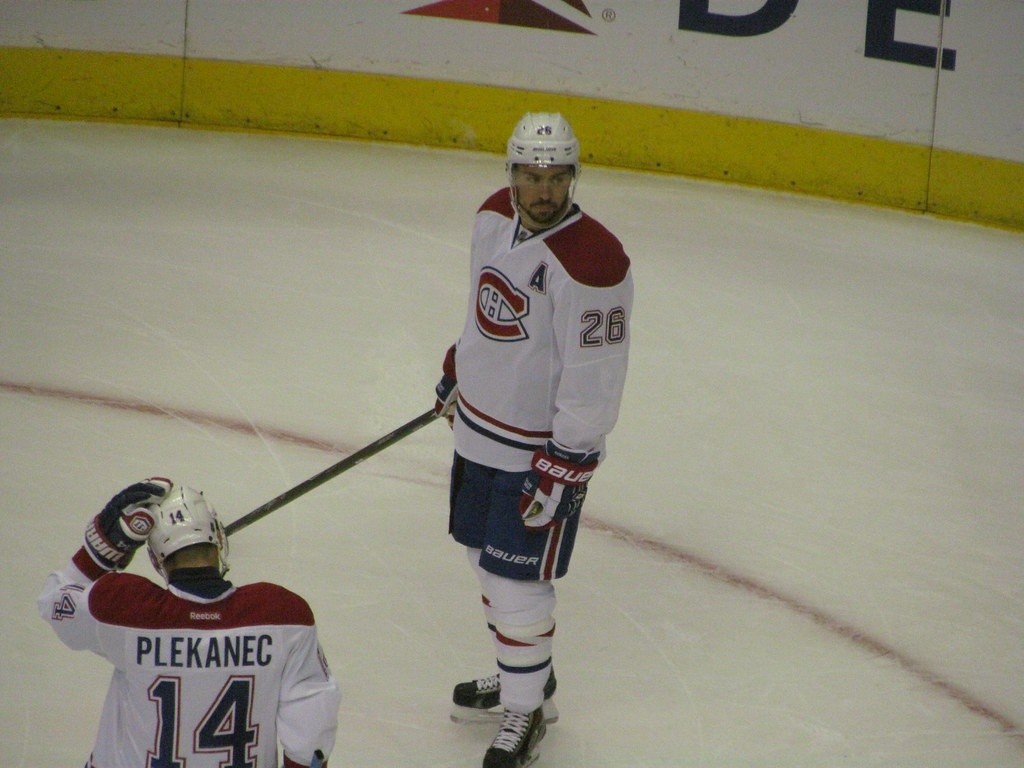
[482,704,547,768]
[449,666,559,726]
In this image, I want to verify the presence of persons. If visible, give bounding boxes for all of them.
[36,476,340,768]
[434,110,633,768]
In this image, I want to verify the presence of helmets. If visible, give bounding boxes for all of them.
[505,111,581,182]
[146,485,223,562]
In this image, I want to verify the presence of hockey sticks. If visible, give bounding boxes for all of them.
[224,407,439,538]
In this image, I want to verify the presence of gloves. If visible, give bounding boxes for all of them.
[519,435,601,532]
[83,476,173,571]
[435,344,459,430]
